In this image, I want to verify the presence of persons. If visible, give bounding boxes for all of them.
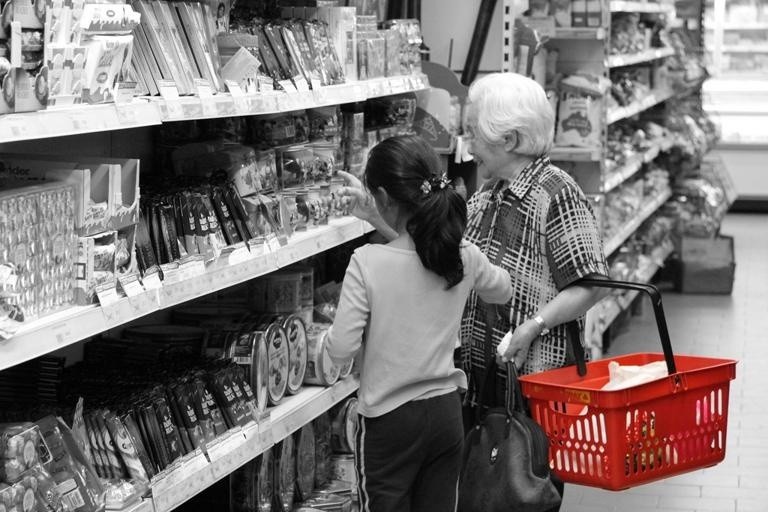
[323,133,514,512]
[336,72,612,512]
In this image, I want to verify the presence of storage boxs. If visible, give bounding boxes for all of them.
[674,230,738,297]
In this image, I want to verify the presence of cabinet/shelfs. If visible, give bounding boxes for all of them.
[526,1,682,362]
[699,0,765,210]
[661,13,735,244]
[0,69,464,512]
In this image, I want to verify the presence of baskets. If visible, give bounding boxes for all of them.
[517,350,739,492]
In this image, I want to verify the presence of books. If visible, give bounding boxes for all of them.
[124,0,359,97]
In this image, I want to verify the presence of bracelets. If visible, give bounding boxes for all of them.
[531,312,550,337]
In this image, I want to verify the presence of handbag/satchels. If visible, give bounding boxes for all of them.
[455,407,562,512]
